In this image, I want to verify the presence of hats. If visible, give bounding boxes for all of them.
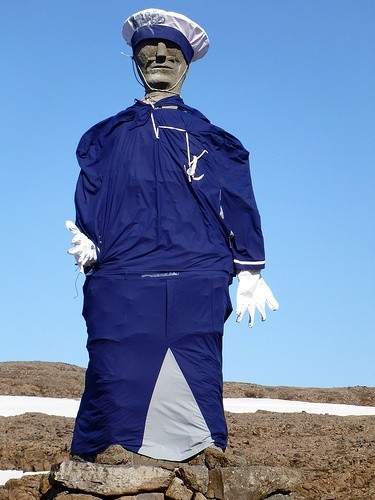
[122,10,210,65]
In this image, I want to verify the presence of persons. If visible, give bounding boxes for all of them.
[65,9,278,461]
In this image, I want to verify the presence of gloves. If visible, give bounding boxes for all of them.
[234,270,278,326]
[62,219,97,270]
